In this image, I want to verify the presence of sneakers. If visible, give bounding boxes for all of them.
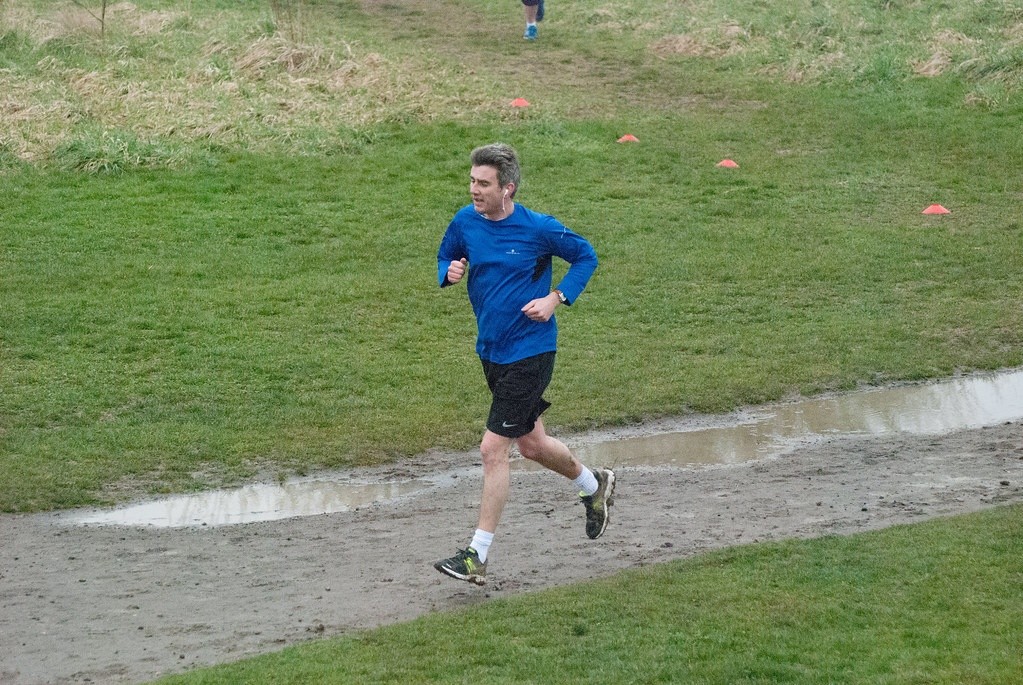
[432,545,488,586]
[522,25,538,41]
[535,0,545,20]
[577,468,616,540]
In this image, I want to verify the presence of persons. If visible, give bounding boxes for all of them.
[433,143,615,586]
[520,0,545,40]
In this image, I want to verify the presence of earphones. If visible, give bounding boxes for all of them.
[504,188,508,195]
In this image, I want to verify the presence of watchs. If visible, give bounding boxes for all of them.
[552,289,566,302]
